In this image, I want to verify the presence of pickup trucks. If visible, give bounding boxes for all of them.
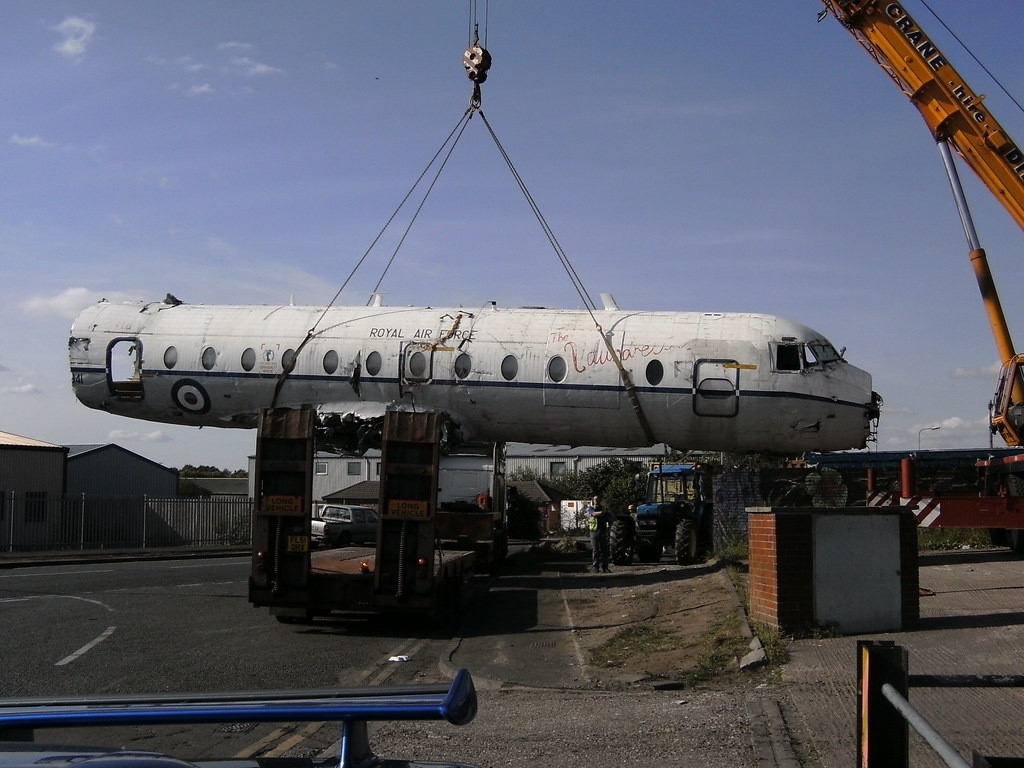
[311,504,379,549]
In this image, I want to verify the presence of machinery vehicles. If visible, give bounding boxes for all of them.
[610,462,715,566]
[465,0,1024,556]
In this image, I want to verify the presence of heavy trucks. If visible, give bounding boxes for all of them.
[248,406,507,625]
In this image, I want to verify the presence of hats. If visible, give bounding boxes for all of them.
[628,505,635,510]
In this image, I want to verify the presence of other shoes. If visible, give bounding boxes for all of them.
[594,567,599,573]
[603,569,611,573]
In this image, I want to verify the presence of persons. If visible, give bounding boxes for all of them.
[628,505,638,555]
[589,496,612,573]
